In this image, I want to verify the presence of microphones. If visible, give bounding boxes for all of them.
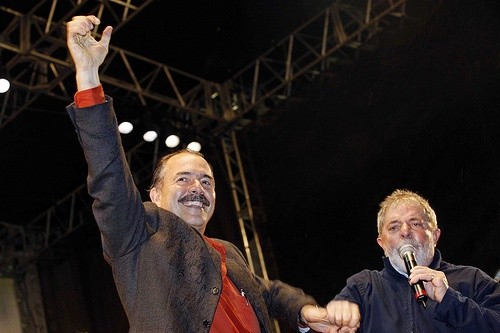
[400,245,429,308]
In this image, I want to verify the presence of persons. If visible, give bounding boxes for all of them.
[325,188,500,333]
[66,15,357,333]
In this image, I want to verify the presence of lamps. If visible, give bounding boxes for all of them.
[115,111,202,152]
[0,66,12,94]
